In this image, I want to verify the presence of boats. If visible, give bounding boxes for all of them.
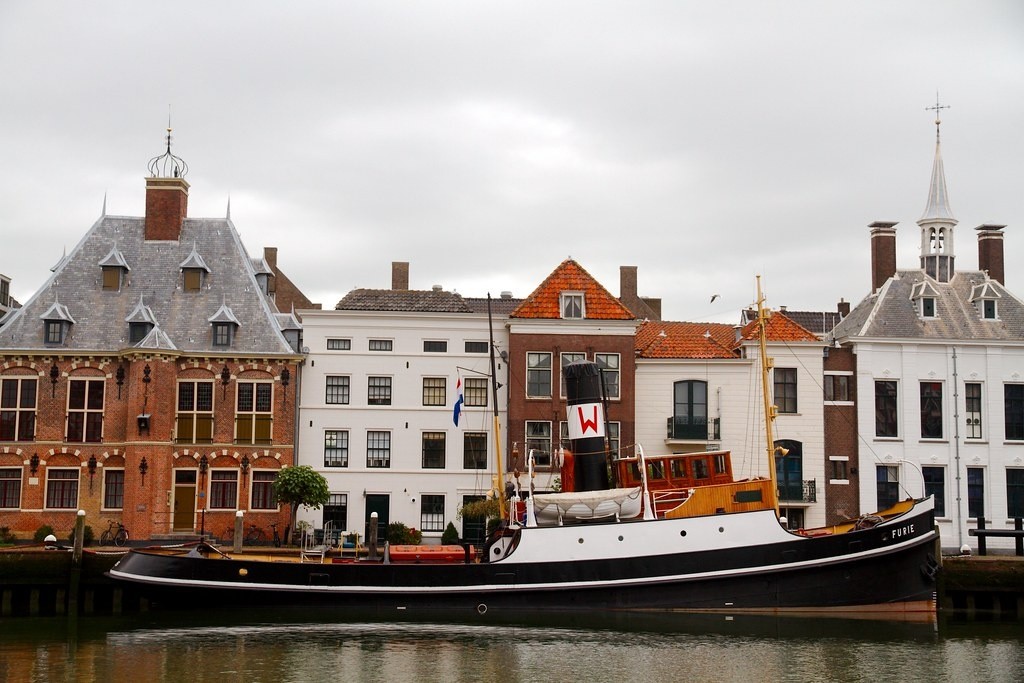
[101,270,942,611]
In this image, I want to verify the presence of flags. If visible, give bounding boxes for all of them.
[453,369,464,426]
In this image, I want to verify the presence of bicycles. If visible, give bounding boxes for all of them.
[267,525,282,548]
[221,521,266,546]
[98,520,129,547]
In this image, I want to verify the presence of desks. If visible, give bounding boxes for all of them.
[344,536,362,547]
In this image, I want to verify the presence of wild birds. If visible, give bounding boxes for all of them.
[710,294,722,305]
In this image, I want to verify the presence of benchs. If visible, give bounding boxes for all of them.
[313,529,342,544]
